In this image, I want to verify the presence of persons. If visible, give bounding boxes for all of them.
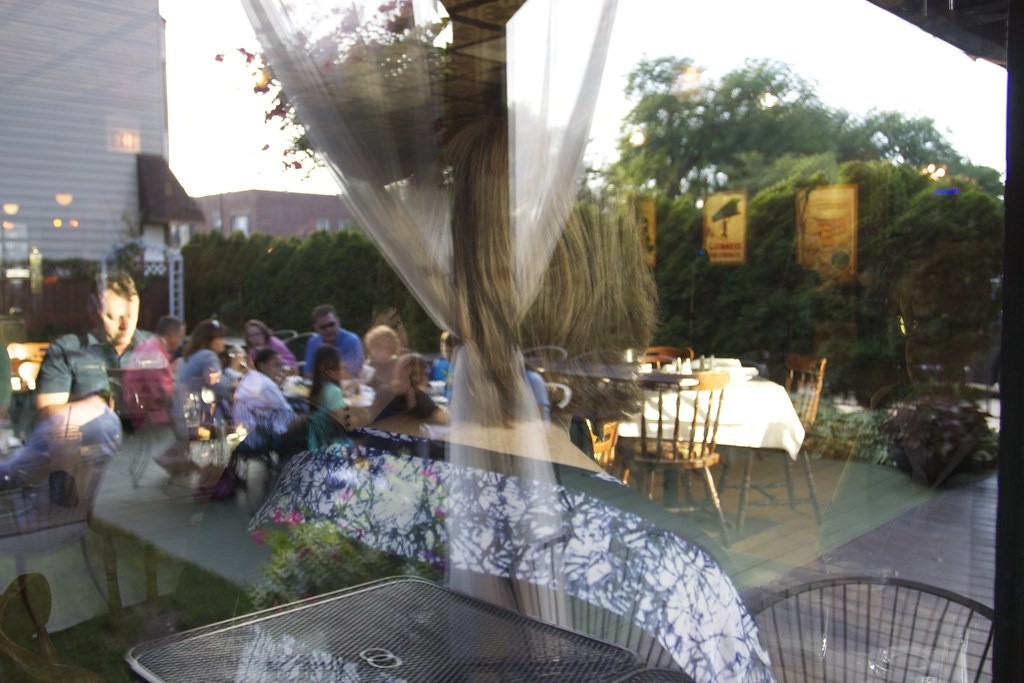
[35,270,190,467]
[0,341,13,418]
[121,303,551,451]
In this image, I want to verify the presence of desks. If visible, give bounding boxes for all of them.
[613,667,696,683]
[121,573,636,683]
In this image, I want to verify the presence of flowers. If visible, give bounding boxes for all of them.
[214,0,468,181]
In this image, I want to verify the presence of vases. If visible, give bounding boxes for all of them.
[312,108,441,195]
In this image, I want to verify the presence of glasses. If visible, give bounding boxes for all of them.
[318,320,337,330]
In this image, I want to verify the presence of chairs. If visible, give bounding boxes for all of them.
[0,337,998,683]
